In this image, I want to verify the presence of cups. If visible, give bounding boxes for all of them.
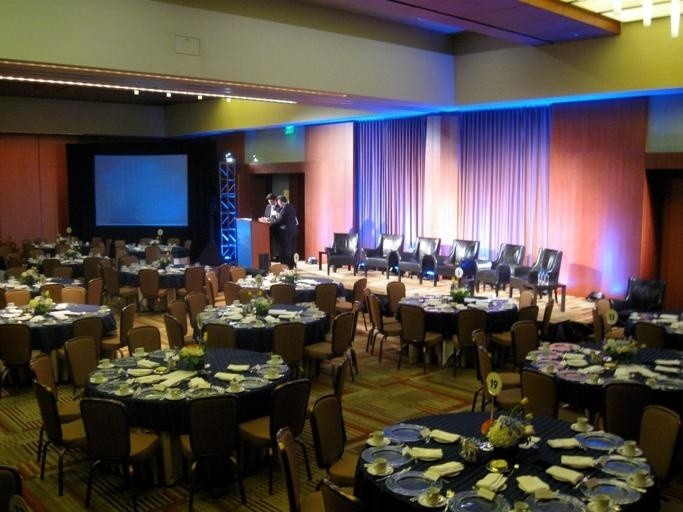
[631,470,648,485]
[119,384,128,393]
[426,487,441,505]
[271,354,280,366]
[170,387,180,398]
[230,381,239,391]
[93,373,104,380]
[587,493,610,512]
[367,430,385,442]
[100,359,109,367]
[267,370,277,378]
[620,440,636,454]
[372,458,387,474]
[134,347,145,356]
[590,374,597,382]
[576,416,587,430]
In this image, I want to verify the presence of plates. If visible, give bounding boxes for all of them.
[367,466,393,477]
[557,369,582,382]
[267,361,283,367]
[597,455,651,479]
[163,393,187,400]
[111,357,136,369]
[132,352,149,359]
[96,382,118,394]
[113,390,133,396]
[649,375,682,390]
[186,385,225,400]
[416,494,446,508]
[584,378,604,386]
[523,493,587,512]
[148,350,176,360]
[224,386,243,394]
[135,388,166,400]
[263,376,281,380]
[446,490,512,512]
[580,479,640,505]
[89,377,107,385]
[96,364,113,369]
[525,348,559,361]
[384,470,439,498]
[615,448,643,456]
[384,424,428,445]
[574,431,624,451]
[626,478,654,488]
[569,424,593,433]
[95,368,128,381]
[531,361,563,373]
[361,446,417,470]
[368,436,391,448]
[239,379,266,389]
[549,342,580,354]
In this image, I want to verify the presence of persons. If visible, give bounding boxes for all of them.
[270,195,297,266]
[264,193,283,262]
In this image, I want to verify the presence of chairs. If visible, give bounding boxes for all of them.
[238,375,314,499]
[217,262,234,290]
[41,258,61,276]
[87,279,106,305]
[276,427,357,512]
[539,299,556,338]
[30,353,81,461]
[269,321,306,362]
[205,270,226,307]
[119,255,140,266]
[518,368,560,420]
[62,332,100,402]
[137,269,168,314]
[83,256,102,280]
[79,396,163,511]
[517,304,540,326]
[0,323,42,397]
[238,287,265,306]
[269,282,296,307]
[430,239,480,288]
[609,274,668,330]
[597,379,629,438]
[591,307,606,345]
[126,323,161,358]
[366,292,404,364]
[636,403,682,481]
[173,390,252,512]
[395,237,441,285]
[60,286,86,307]
[163,314,186,348]
[224,281,242,306]
[326,232,356,275]
[451,307,491,379]
[39,284,64,301]
[145,246,161,264]
[54,266,72,278]
[361,233,404,279]
[270,264,289,274]
[472,329,488,383]
[397,301,445,373]
[166,299,188,337]
[518,289,536,312]
[472,346,524,417]
[305,309,356,389]
[230,266,245,282]
[5,268,22,282]
[57,315,104,385]
[473,244,526,292]
[183,264,205,294]
[186,292,211,332]
[309,393,360,487]
[100,302,137,359]
[335,277,370,332]
[319,475,363,512]
[385,281,407,321]
[632,321,666,350]
[199,323,237,349]
[4,288,30,309]
[595,298,613,324]
[313,282,339,312]
[104,267,138,305]
[32,381,86,496]
[509,246,563,304]
[54,244,71,258]
[509,317,541,368]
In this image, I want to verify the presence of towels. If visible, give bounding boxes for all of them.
[476,472,508,501]
[224,311,238,315]
[55,314,69,321]
[515,475,550,494]
[226,364,250,371]
[49,310,71,315]
[545,464,583,485]
[278,311,300,318]
[54,303,68,310]
[661,309,683,331]
[214,371,242,381]
[578,364,605,376]
[411,446,443,458]
[429,428,461,444]
[560,454,593,467]
[229,314,243,320]
[136,359,160,368]
[127,368,153,376]
[564,351,585,360]
[548,438,579,448]
[565,359,588,367]
[422,460,465,482]
[655,358,681,365]
[159,369,198,387]
[654,366,680,375]
[268,308,287,314]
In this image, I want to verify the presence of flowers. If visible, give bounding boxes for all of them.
[248,297,274,310]
[177,341,204,359]
[450,276,469,297]
[487,395,532,448]
[28,289,58,309]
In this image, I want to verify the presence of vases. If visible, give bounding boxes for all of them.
[255,307,270,318]
[602,334,648,359]
[34,305,47,316]
[452,293,467,304]
[177,352,205,371]
